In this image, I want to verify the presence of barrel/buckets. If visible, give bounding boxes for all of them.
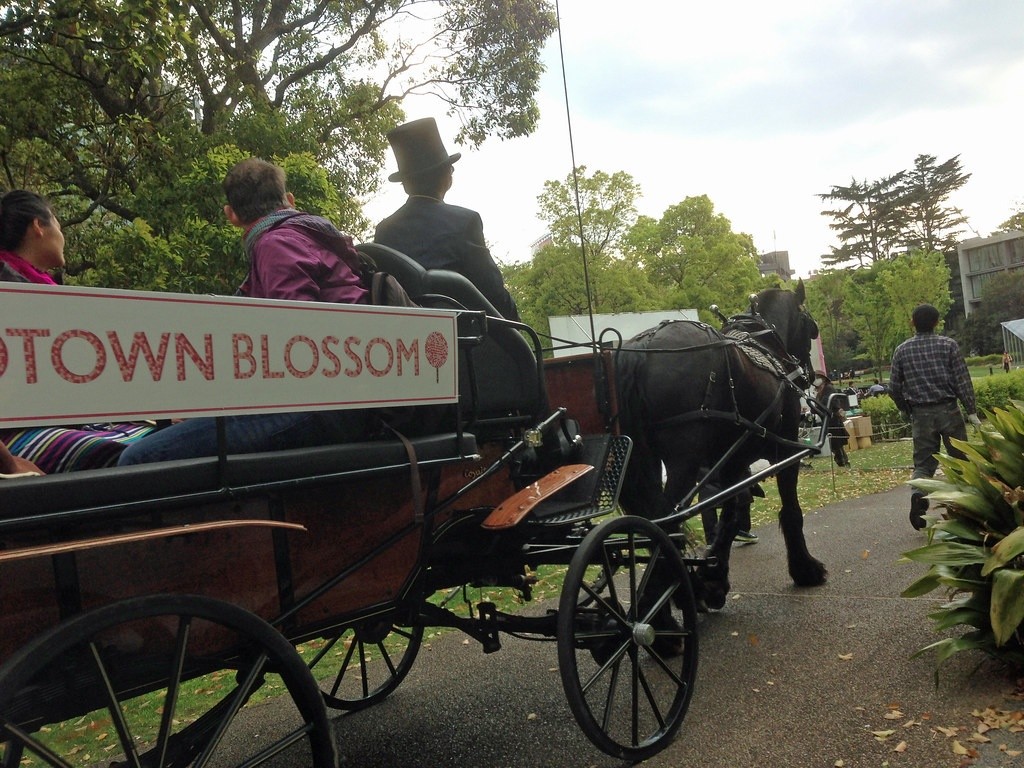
[809,427,832,457]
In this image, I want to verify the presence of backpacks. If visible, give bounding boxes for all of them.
[364,252,422,308]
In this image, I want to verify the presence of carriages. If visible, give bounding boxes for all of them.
[0,274,849,768]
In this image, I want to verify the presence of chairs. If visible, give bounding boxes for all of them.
[834,383,889,406]
[352,242,543,404]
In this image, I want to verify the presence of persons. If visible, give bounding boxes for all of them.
[697,461,765,549]
[374,118,581,477]
[864,379,884,397]
[810,370,842,426]
[889,305,982,531]
[0,189,156,476]
[848,382,862,399]
[1002,352,1012,373]
[117,159,367,466]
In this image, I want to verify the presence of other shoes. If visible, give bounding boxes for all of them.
[909,492,927,531]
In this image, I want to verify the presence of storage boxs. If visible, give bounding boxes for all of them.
[808,416,875,457]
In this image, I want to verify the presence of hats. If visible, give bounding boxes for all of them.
[388,117,461,183]
[812,370,830,386]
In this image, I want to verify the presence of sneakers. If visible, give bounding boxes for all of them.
[735,531,758,542]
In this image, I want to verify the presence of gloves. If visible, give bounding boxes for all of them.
[969,413,981,434]
[900,410,911,424]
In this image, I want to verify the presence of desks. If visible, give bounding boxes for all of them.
[858,387,870,394]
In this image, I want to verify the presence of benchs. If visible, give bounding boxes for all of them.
[0,432,482,533]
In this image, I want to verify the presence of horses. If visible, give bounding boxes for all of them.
[606,279,829,654]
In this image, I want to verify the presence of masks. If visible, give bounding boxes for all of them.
[813,377,823,387]
